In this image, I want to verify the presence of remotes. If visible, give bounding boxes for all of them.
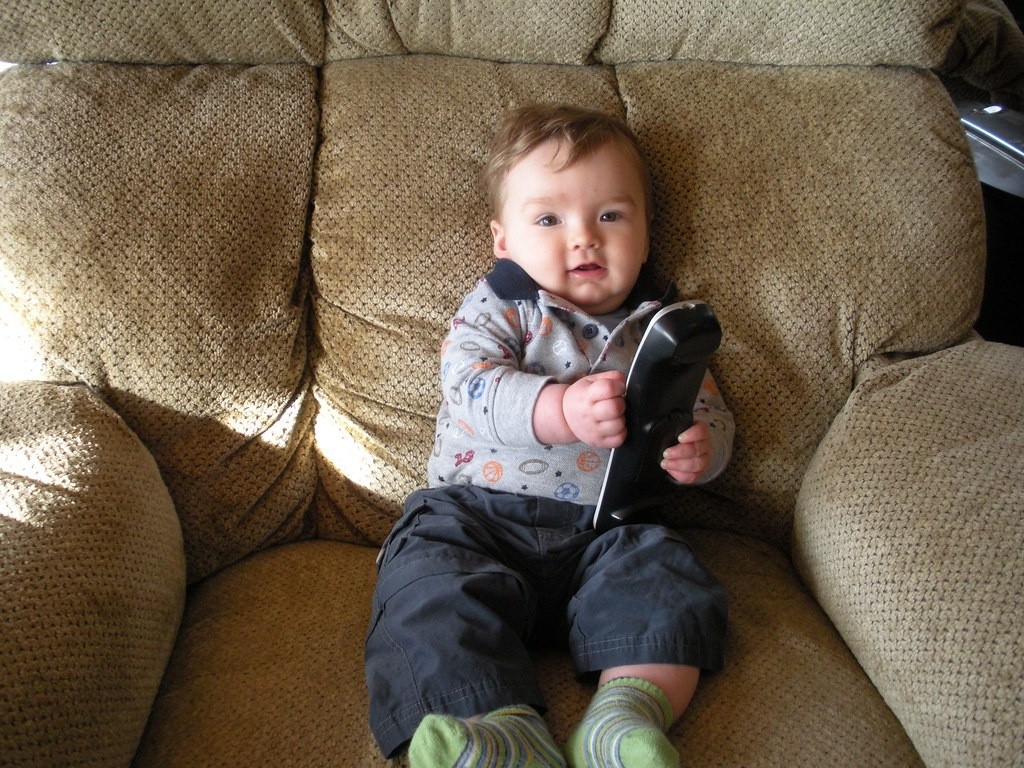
[594,299,722,531]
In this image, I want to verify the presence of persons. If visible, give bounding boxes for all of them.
[363,101,737,768]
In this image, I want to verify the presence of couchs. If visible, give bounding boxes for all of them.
[0,0,1024,768]
